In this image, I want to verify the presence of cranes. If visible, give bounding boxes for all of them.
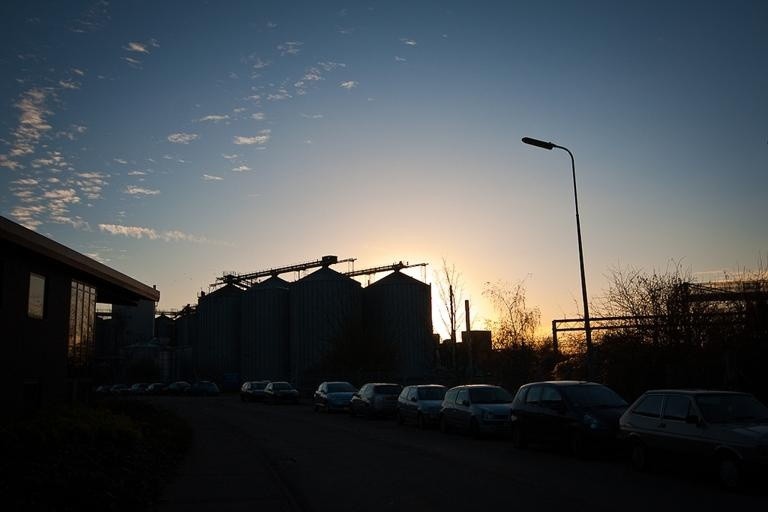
[211,257,427,289]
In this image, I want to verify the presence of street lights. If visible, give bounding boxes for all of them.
[523,137,593,355]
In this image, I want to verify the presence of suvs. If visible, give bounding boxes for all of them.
[509,381,633,445]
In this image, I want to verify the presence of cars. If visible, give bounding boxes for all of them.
[442,384,513,438]
[394,383,450,425]
[316,382,356,413]
[619,388,768,483]
[241,379,299,402]
[348,382,404,416]
[94,382,220,397]
